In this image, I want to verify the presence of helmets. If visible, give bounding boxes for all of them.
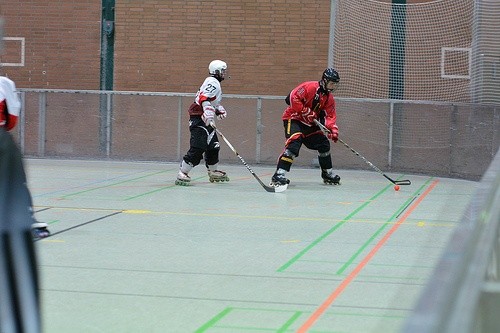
[208,60,227,78]
[321,67,340,92]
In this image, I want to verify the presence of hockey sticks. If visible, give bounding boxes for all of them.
[313,118,411,185]
[210,119,289,193]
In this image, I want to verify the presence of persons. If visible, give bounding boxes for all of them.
[173,60,229,187]
[268,68,340,186]
[0,77,50,238]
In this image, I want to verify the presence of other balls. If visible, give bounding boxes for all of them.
[394,185,399,191]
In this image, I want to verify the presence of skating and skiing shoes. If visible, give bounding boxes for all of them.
[207,162,230,183]
[175,160,192,186]
[30,215,50,237]
[321,169,341,185]
[270,172,290,188]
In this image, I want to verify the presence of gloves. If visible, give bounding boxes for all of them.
[326,125,339,143]
[300,107,317,124]
[215,105,227,120]
[200,106,215,126]
